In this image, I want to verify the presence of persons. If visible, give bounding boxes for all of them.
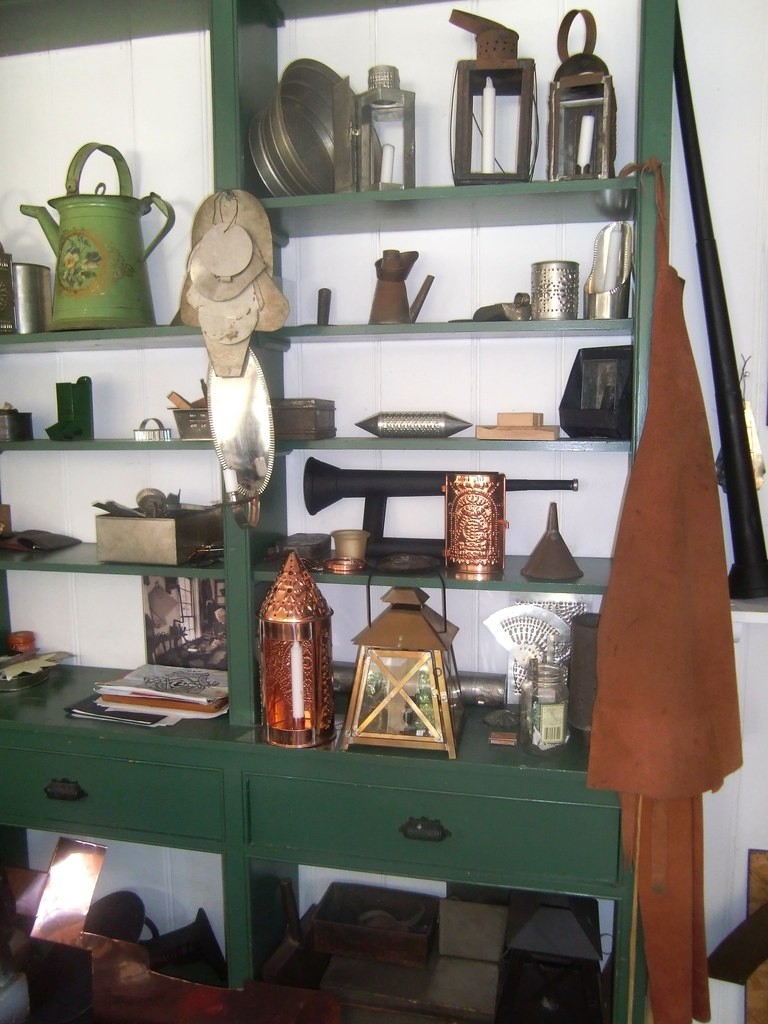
[213,608,227,647]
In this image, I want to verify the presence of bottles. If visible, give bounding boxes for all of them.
[517,664,571,757]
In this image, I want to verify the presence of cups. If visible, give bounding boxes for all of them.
[10,262,54,336]
[330,529,372,563]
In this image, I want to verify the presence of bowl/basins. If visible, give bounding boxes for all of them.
[249,57,382,197]
[167,407,213,442]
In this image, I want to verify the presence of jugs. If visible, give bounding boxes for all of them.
[20,142,174,333]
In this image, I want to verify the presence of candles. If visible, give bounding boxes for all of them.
[575,108,597,175]
[601,220,623,292]
[480,76,496,174]
[375,144,396,184]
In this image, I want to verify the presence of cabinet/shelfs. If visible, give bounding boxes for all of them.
[0,2,247,1024]
[228,0,684,1020]
